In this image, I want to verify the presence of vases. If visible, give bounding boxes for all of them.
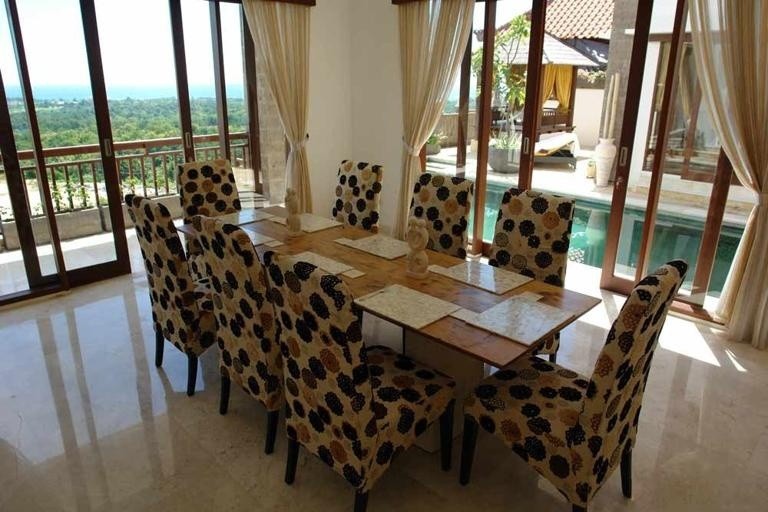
[594,138,617,189]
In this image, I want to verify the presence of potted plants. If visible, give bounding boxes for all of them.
[472,14,531,173]
